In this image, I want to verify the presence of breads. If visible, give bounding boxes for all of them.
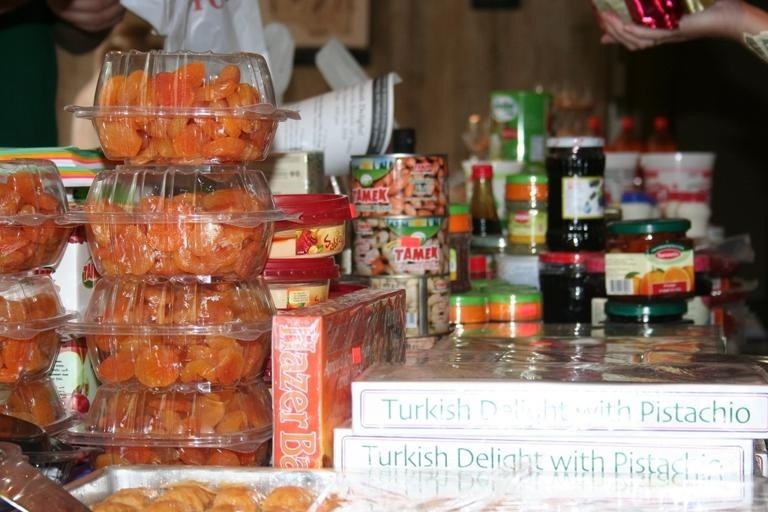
[90,482,313,512]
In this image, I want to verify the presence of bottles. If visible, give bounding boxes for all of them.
[468,163,501,236]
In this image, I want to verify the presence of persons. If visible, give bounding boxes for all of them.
[0,1,128,34]
[595,1,768,68]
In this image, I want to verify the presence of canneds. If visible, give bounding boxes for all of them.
[348,152,473,339]
[488,89,696,323]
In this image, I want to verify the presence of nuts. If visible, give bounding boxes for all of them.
[0,380,65,426]
[0,169,66,383]
[95,445,269,467]
[86,62,273,436]
